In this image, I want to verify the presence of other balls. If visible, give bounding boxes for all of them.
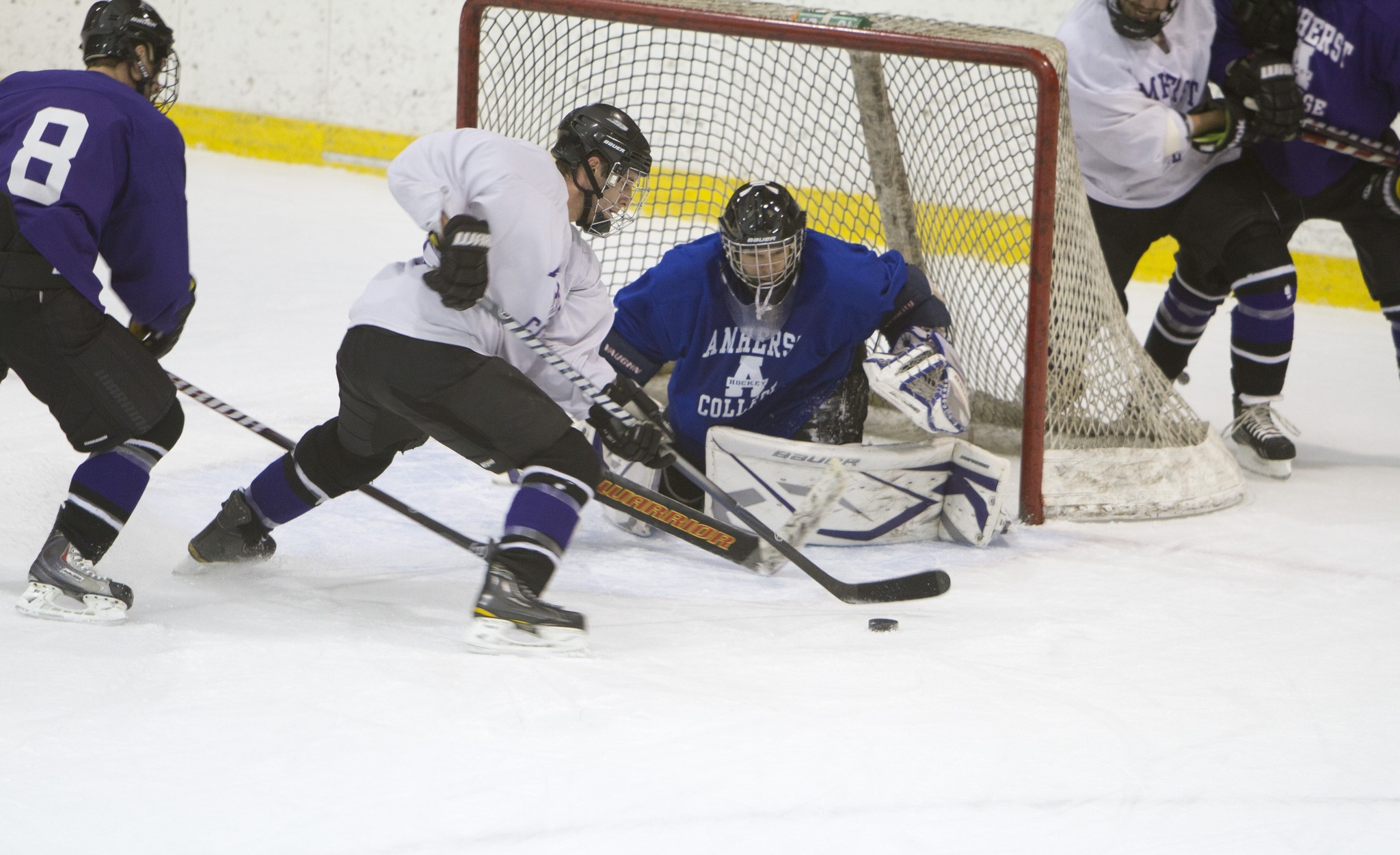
[867,617,899,630]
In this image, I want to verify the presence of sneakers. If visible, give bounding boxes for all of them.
[1220,394,1300,480]
[1011,365,1085,416]
[14,503,134,623]
[171,491,276,574]
[464,538,586,657]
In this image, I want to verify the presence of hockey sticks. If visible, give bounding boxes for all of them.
[422,238,951,606]
[594,459,850,576]
[164,368,499,563]
[1205,78,1400,170]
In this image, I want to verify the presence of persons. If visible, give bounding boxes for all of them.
[1046,0,1298,478]
[1123,0,1400,455]
[0,0,196,627]
[491,180,1010,549]
[170,103,677,657]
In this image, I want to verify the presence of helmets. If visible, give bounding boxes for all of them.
[717,180,807,289]
[79,0,180,116]
[551,104,652,238]
[1107,0,1179,40]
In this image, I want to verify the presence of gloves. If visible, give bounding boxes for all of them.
[423,215,491,311]
[1187,97,1264,155]
[585,372,677,470]
[129,274,196,360]
[1356,166,1400,224]
[1229,55,1303,146]
